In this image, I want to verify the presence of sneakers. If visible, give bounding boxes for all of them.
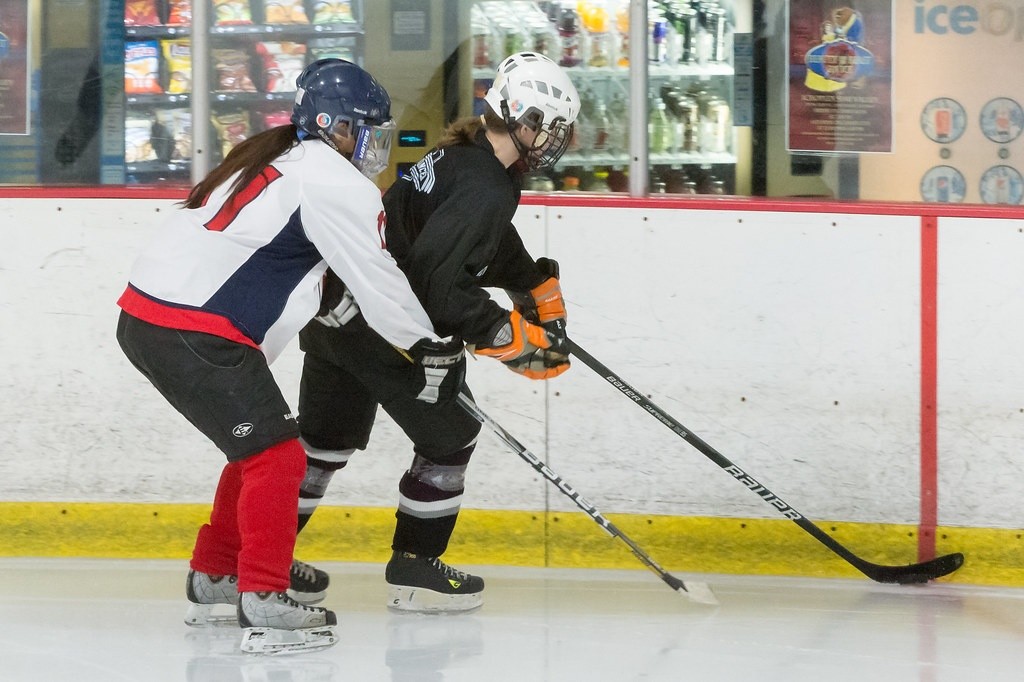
[184,568,242,626]
[287,557,331,601]
[238,591,340,654]
[385,548,485,610]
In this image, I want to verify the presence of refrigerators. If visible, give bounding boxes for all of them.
[456,0,739,198]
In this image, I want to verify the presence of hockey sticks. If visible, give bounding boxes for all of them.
[567,339,964,583]
[391,344,719,605]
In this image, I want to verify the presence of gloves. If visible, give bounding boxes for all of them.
[312,280,370,334]
[474,309,571,378]
[505,257,567,336]
[410,338,467,412]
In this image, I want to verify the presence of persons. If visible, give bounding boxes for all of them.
[293,47,581,619]
[116,58,470,658]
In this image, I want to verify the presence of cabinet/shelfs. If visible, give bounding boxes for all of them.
[453,0,755,197]
[98,0,365,192]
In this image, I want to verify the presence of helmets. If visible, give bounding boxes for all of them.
[487,49,582,175]
[291,56,397,178]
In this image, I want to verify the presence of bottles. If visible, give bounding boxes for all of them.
[650,161,730,195]
[474,80,493,115]
[471,0,630,68]
[648,1,731,66]
[650,82,731,153]
[522,167,626,194]
[568,80,626,155]
